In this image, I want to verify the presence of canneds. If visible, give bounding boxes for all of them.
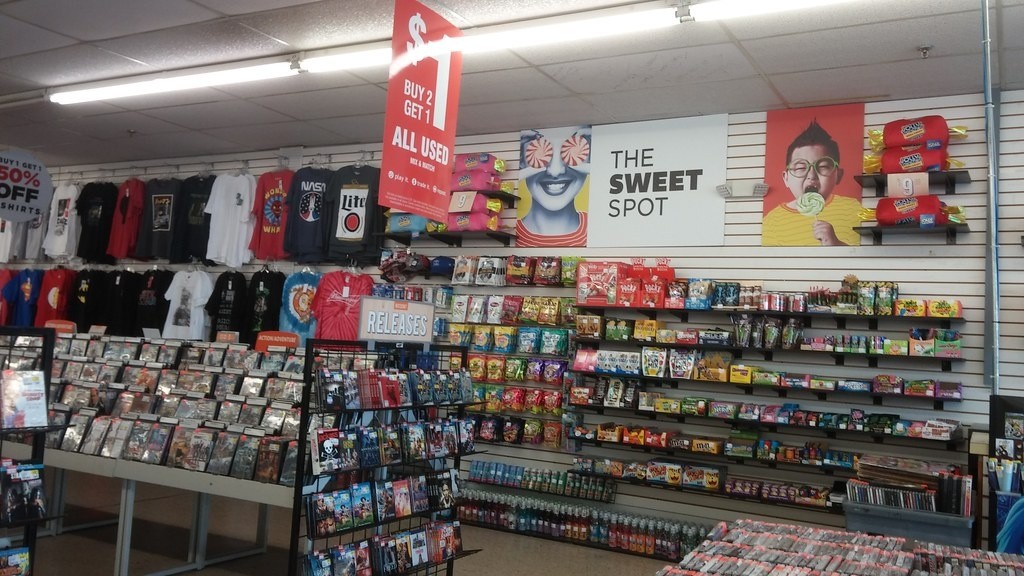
[761,292,806,311]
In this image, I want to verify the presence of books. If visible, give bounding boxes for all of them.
[307,366,475,576]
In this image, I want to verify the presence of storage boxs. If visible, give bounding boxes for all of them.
[575,261,963,507]
[844,490,976,548]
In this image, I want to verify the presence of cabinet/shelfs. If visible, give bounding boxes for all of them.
[371,186,521,248]
[564,305,967,516]
[288,337,489,576]
[0,324,76,575]
[852,169,971,244]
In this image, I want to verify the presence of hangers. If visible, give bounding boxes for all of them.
[0,148,370,277]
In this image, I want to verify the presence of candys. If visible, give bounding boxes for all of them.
[870,116,969,150]
[797,193,824,215]
[863,147,964,171]
[858,197,968,225]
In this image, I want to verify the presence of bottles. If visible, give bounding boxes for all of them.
[456,488,713,560]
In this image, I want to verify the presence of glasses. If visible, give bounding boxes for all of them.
[786,157,840,178]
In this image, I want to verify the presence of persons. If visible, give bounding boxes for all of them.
[440,482,456,508]
[516,125,592,247]
[763,117,862,247]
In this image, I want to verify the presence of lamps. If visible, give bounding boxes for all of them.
[0,2,709,109]
